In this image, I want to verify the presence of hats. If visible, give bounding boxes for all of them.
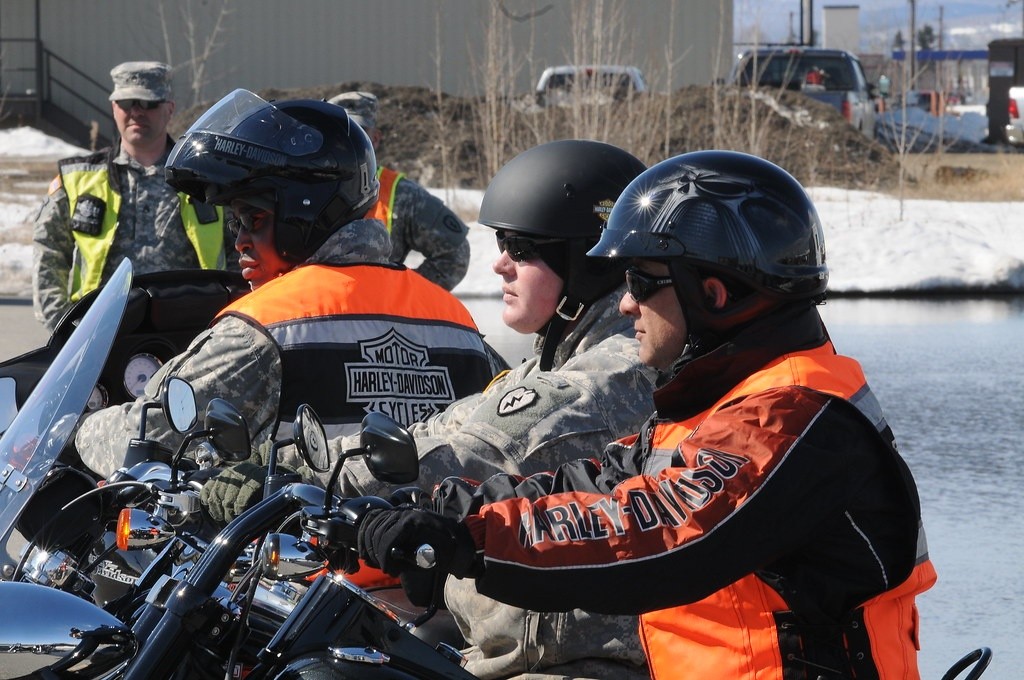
[108,60,178,102]
[326,91,381,129]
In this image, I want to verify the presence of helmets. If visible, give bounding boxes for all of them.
[585,151,825,301]
[478,139,648,242]
[163,87,380,264]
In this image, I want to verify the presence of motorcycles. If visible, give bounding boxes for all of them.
[0,257,993,680]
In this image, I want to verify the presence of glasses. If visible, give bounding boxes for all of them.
[624,264,674,303]
[493,229,560,263]
[226,205,267,240]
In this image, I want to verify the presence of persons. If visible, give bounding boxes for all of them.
[326,92,471,291]
[32,60,243,335]
[50,90,938,680]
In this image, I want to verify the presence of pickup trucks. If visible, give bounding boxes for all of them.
[534,65,650,105]
[710,48,892,142]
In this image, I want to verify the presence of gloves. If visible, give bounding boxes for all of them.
[357,503,478,580]
[200,460,301,523]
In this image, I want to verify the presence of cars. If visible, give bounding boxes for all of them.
[1003,84,1024,147]
